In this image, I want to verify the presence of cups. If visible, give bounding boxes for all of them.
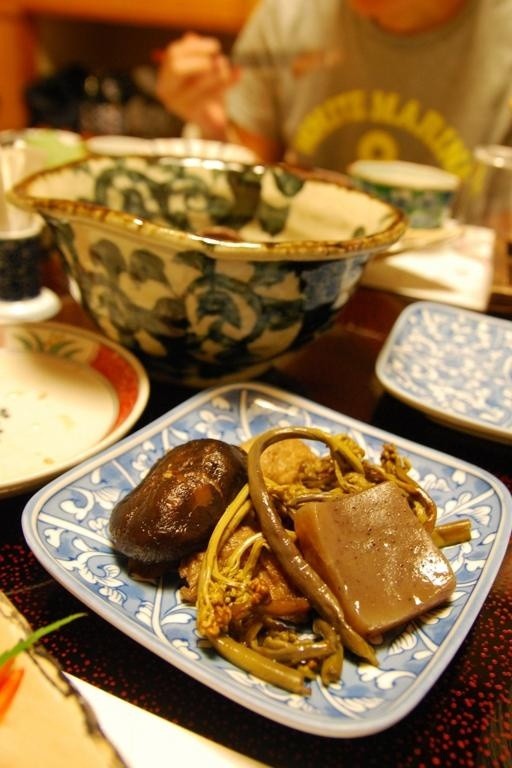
[469,141,512,293]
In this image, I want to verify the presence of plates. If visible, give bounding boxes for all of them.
[2,320,151,497]
[375,300,510,437]
[21,378,510,746]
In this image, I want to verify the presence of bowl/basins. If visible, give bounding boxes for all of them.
[10,153,404,380]
[353,159,462,230]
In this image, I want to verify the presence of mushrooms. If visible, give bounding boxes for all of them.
[110,438,248,566]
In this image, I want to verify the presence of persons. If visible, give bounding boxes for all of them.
[155,0,512,193]
